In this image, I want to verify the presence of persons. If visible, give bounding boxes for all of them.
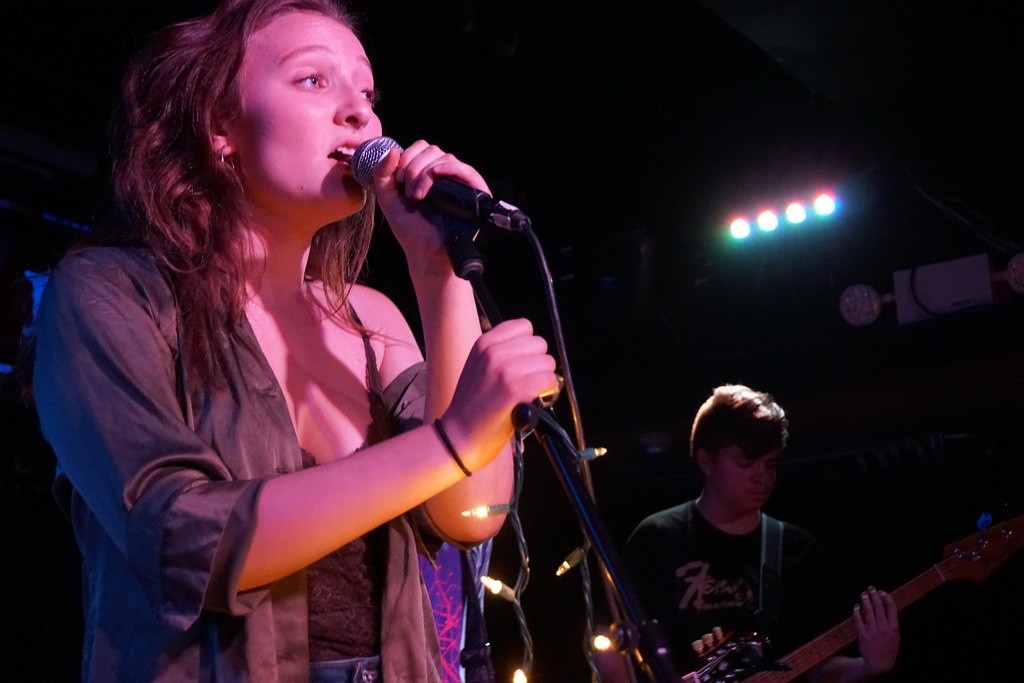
[32,0,562,683]
[588,383,901,683]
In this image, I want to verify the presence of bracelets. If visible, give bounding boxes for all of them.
[435,417,472,477]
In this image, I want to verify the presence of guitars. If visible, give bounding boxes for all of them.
[740,513,1024,683]
[677,625,789,683]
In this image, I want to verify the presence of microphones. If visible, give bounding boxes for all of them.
[350,136,531,234]
